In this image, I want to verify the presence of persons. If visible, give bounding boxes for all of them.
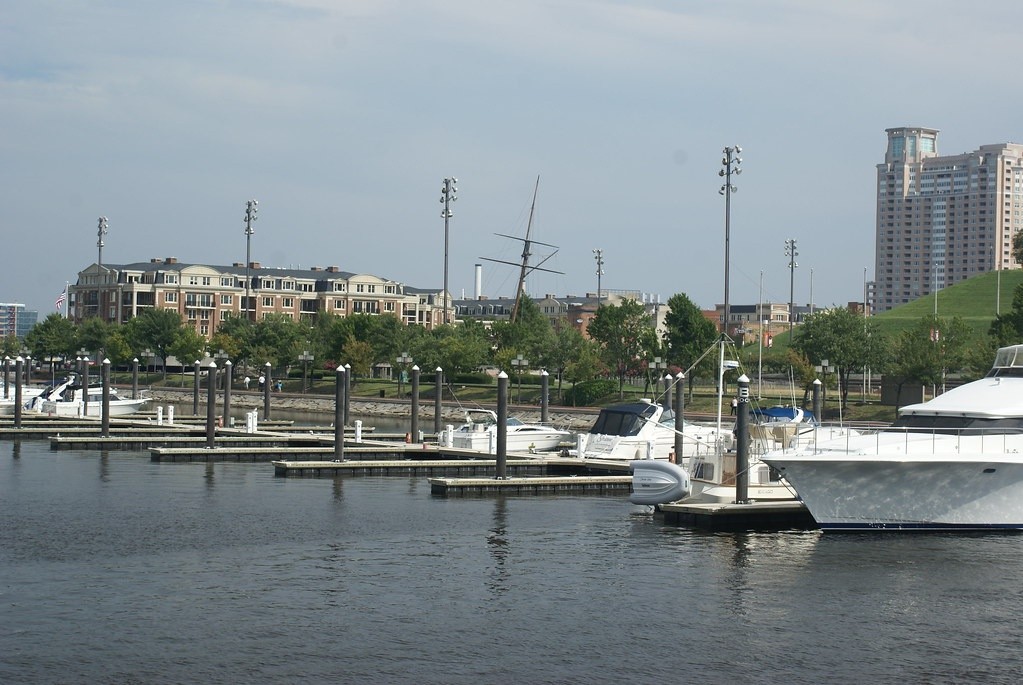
[244,375,250,391]
[730,397,737,415]
[258,373,265,392]
[270,378,282,393]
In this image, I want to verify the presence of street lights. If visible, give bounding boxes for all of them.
[785,238,799,343]
[593,249,605,310]
[440,178,458,325]
[244,200,258,320]
[718,144,743,393]
[96,216,109,318]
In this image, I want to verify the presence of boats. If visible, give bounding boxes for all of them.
[0,375,153,416]
[587,365,820,457]
[628,332,862,528]
[437,410,575,455]
[757,343,1023,534]
